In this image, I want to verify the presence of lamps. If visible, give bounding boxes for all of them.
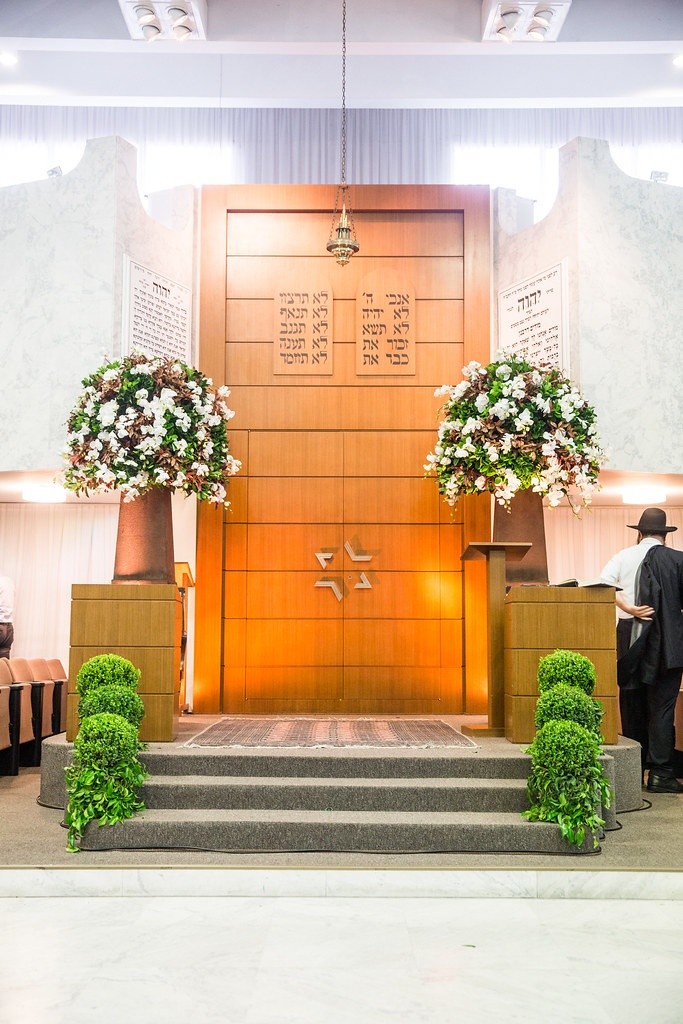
[480,0,573,42]
[118,0,208,42]
[326,0,360,267]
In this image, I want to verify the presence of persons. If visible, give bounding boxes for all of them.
[0,576,15,658]
[601,508,683,794]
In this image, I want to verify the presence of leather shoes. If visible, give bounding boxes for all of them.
[646,772,683,793]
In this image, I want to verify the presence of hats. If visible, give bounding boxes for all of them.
[627,508,677,532]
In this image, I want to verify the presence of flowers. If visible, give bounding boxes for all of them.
[54,351,243,514]
[422,349,609,521]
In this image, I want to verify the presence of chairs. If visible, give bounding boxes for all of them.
[0,657,69,776]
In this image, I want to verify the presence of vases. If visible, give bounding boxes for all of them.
[111,481,175,585]
[491,488,550,586]
